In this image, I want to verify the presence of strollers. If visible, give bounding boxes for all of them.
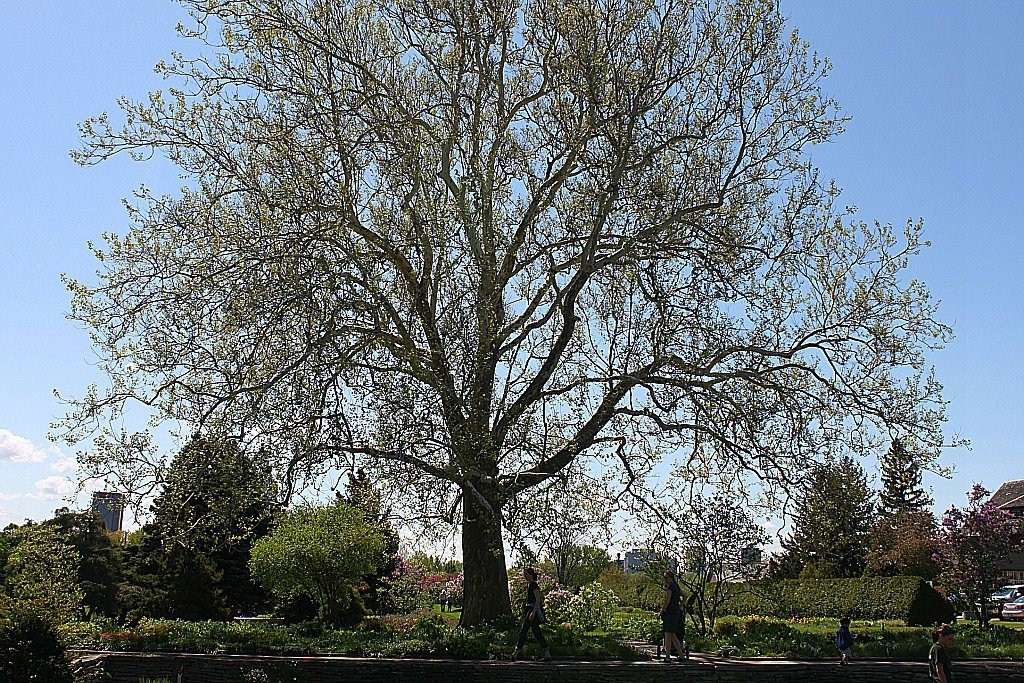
[655,604,690,660]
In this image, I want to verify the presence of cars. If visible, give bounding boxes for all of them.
[947,585,1024,622]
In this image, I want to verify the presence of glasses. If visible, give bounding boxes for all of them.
[662,576,671,580]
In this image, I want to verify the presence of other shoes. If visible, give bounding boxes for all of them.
[537,656,552,663]
[659,658,670,662]
[671,657,686,662]
[502,654,516,662]
[840,660,846,665]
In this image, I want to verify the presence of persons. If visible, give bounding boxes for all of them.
[836,617,854,666]
[928,623,955,682]
[447,595,453,612]
[440,589,447,612]
[660,569,688,664]
[505,568,552,662]
